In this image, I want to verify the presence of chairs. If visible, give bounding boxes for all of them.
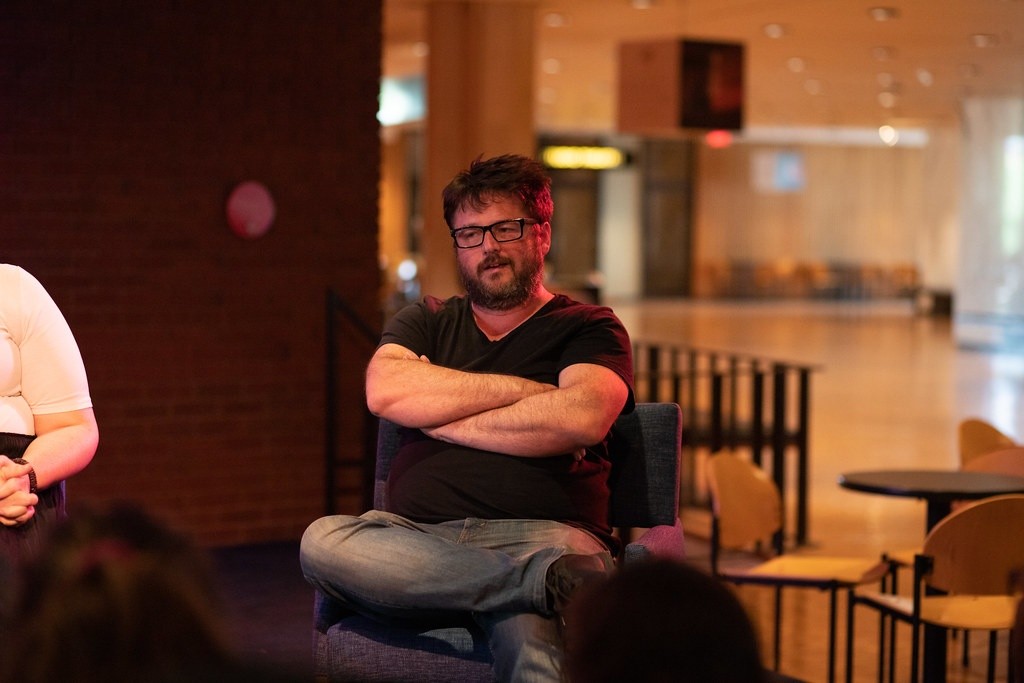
[312,402,684,683]
[704,449,897,683]
[878,447,1024,683]
[849,492,1024,683]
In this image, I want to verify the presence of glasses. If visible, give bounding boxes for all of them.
[450,218,541,249]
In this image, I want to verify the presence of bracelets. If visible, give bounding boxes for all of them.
[13,458,38,493]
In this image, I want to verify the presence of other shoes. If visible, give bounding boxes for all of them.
[546,551,616,651]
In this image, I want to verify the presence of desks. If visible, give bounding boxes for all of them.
[839,468,1024,683]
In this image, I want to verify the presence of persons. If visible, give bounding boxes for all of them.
[557,553,808,683]
[0,262,99,571]
[299,156,634,683]
[0,500,259,682]
